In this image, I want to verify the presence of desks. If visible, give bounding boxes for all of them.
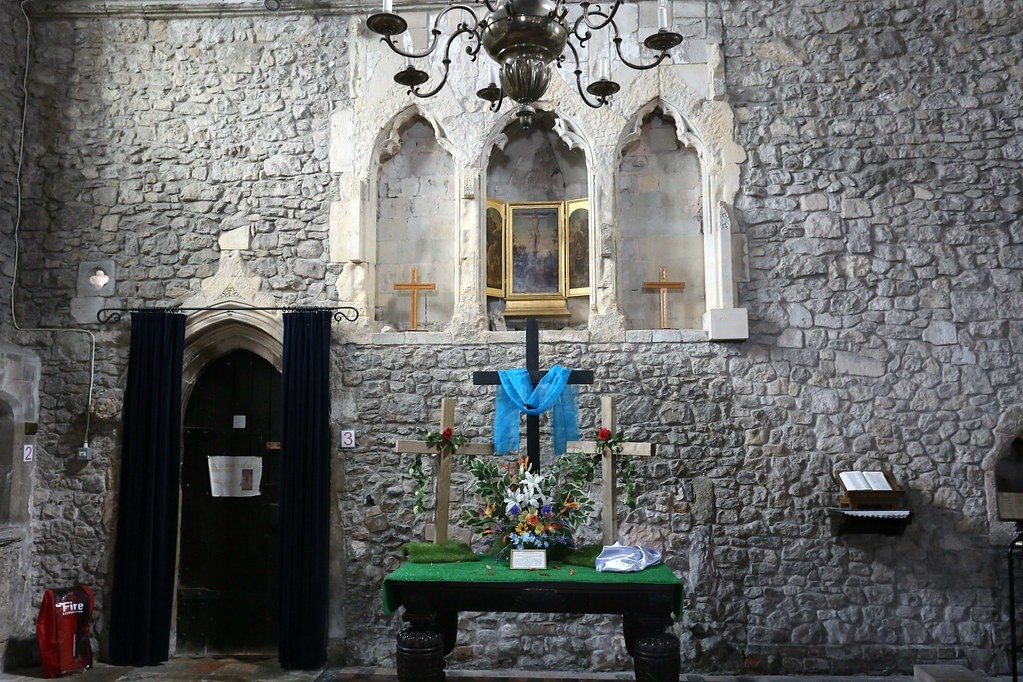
[382,556,685,681]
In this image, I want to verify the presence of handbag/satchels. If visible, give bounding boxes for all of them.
[36,584,95,678]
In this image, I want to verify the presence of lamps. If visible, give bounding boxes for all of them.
[365,0,682,129]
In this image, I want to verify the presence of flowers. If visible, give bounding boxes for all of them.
[411,423,642,560]
